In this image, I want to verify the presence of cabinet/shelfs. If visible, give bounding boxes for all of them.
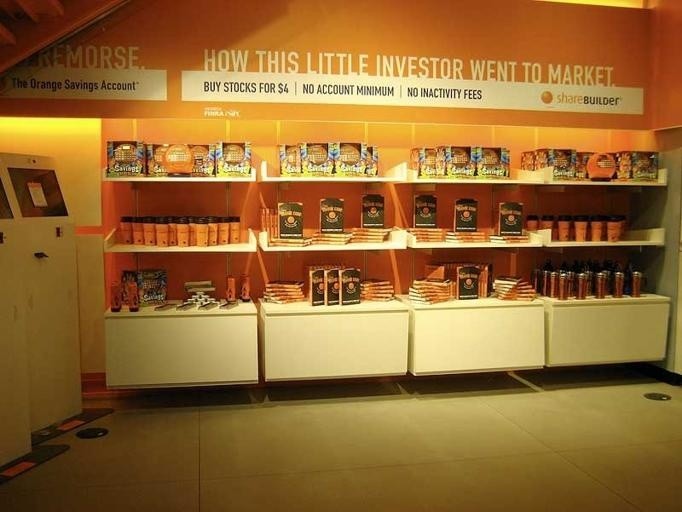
[102,160,671,390]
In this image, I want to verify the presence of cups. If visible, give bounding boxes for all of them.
[531,269,641,300]
[526,215,626,242]
[120,216,240,247]
